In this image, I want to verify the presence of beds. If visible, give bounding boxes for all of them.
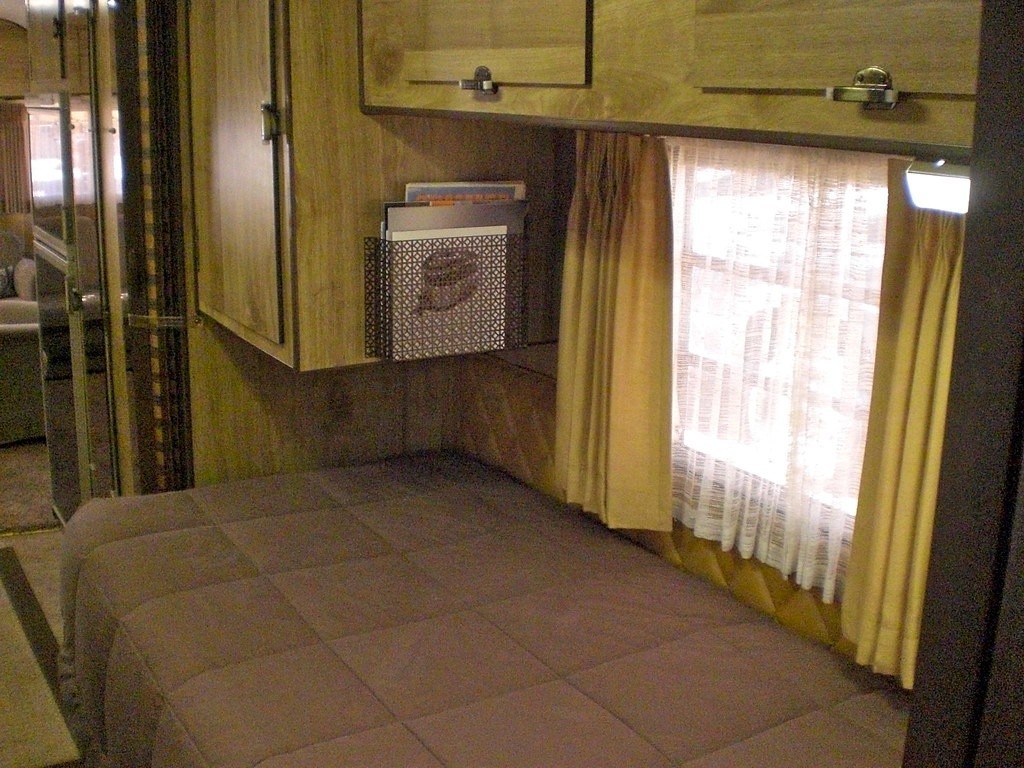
[49,446,910,767]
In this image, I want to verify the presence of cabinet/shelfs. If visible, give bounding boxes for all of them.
[175,0,977,374]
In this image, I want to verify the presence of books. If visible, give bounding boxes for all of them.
[380,180,527,361]
